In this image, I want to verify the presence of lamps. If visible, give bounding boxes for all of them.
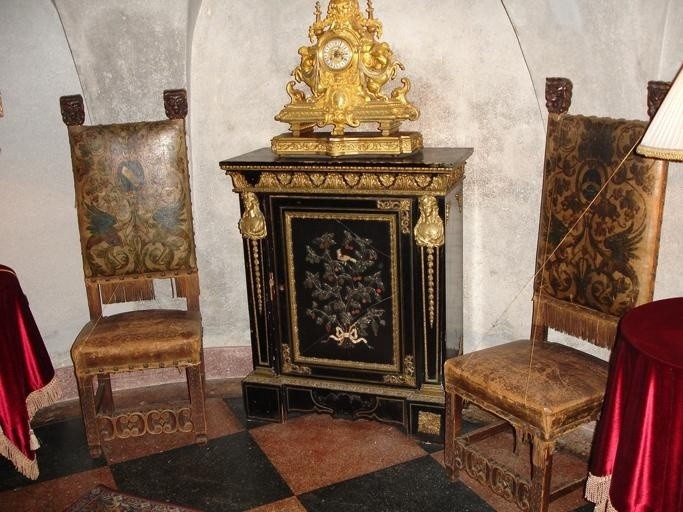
[632,63,683,163]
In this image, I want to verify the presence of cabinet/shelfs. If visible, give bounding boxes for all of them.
[220,147,475,448]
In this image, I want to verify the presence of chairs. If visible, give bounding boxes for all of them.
[442,78,670,510]
[57,86,208,459]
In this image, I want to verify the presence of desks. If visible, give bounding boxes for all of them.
[1,266,58,483]
[576,296,683,512]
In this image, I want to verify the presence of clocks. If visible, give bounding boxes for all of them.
[269,0,423,158]
